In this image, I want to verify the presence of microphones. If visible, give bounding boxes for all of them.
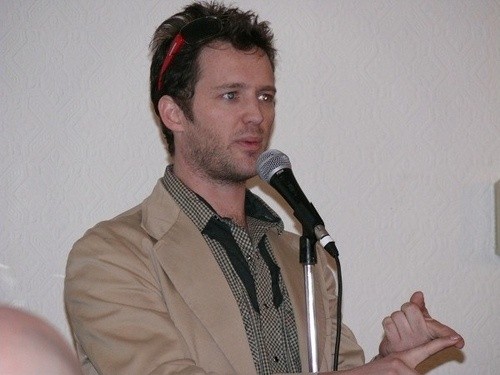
[256,149,339,257]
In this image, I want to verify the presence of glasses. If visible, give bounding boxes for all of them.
[156,15,230,92]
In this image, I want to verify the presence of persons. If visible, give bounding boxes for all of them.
[62,0,466,375]
[0,307,83,375]
